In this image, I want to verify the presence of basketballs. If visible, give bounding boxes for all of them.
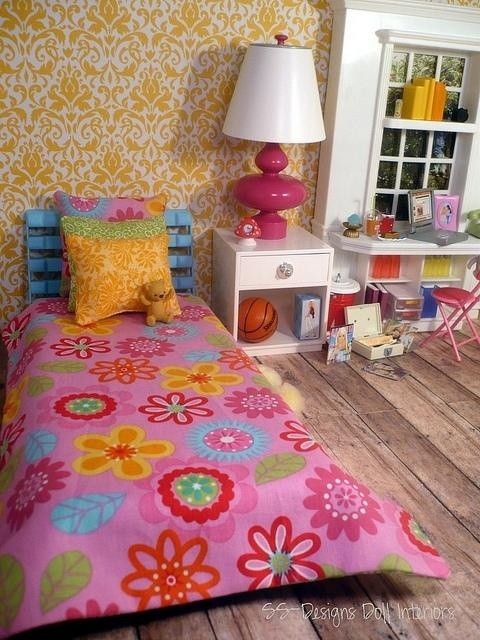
[238,298,277,343]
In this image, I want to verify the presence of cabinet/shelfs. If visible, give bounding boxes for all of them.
[312,0,479,334]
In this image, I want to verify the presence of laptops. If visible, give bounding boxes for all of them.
[408,189,468,247]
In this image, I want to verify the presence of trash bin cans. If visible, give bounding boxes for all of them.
[326,272,361,331]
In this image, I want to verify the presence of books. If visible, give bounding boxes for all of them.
[403,76,447,120]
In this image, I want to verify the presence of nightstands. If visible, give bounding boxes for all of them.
[211,226,334,358]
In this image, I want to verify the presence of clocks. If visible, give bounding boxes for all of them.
[234,215,262,247]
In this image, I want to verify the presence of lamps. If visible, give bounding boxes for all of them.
[221,34,327,240]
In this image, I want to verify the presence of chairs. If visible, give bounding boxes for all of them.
[419,254,480,363]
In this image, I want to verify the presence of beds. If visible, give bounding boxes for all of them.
[0,206,450,637]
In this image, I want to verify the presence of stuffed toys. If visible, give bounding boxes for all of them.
[140,279,175,327]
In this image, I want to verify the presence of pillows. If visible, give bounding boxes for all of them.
[59,215,181,327]
[54,189,167,317]
